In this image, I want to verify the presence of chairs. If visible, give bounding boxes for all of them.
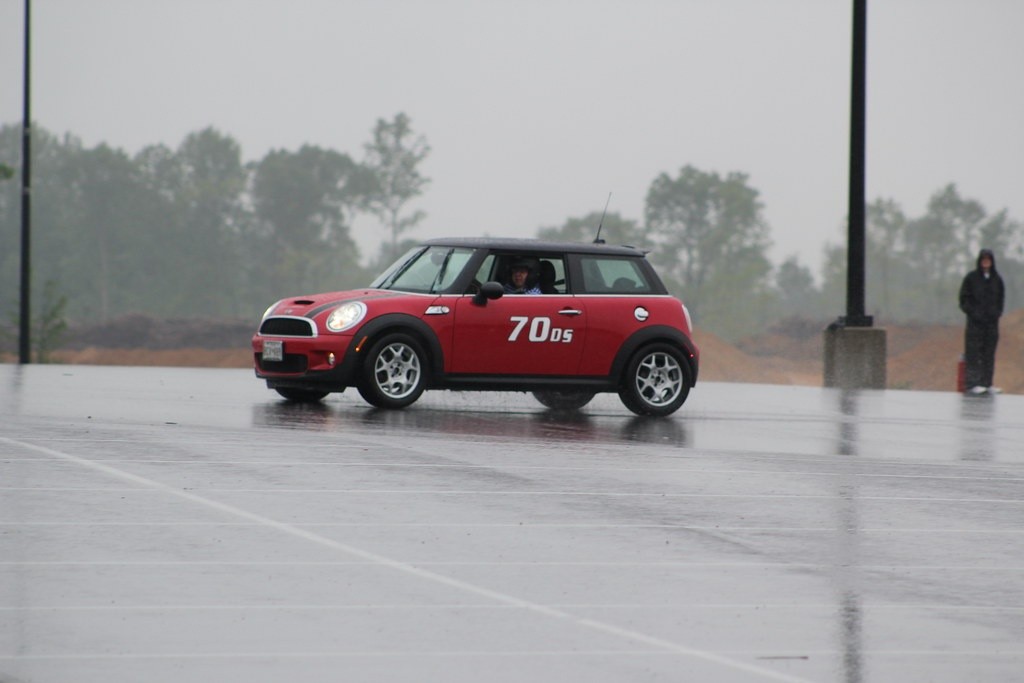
[585,278,635,292]
[532,260,558,293]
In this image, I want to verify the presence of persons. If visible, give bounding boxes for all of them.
[957,248,1005,393]
[504,260,541,294]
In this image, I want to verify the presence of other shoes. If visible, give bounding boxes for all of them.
[988,386,1001,394]
[969,386,987,394]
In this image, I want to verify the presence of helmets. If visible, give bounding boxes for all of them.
[505,251,545,287]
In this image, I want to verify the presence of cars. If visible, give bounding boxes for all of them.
[248,237,702,419]
